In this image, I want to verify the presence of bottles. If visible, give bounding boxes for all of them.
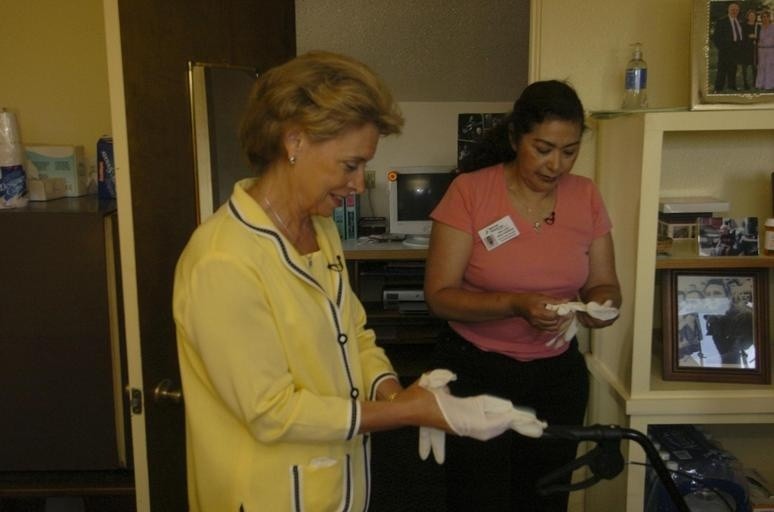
[763,218,774,255]
[0,111,30,209]
[623,41,647,109]
[647,427,740,511]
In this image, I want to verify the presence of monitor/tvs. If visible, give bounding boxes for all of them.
[388,167,455,249]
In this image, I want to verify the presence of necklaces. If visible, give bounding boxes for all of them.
[507,174,556,218]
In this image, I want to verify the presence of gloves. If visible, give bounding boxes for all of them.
[417,366,552,466]
[538,296,618,352]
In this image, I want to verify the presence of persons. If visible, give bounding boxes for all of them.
[711,2,745,94]
[421,77,623,511]
[170,48,552,512]
[755,10,774,90]
[676,275,756,366]
[719,221,732,256]
[741,7,762,91]
[725,217,758,254]
[486,236,496,247]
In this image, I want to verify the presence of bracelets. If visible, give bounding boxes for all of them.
[387,392,399,403]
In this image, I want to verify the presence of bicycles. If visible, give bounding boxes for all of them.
[522,420,738,512]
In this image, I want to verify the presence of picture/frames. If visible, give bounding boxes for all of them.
[658,270,770,383]
[689,1,774,111]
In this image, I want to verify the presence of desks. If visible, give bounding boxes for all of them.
[342,238,430,263]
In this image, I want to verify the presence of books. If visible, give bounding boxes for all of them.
[335,198,347,242]
[346,191,361,240]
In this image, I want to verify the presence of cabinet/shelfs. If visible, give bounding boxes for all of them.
[590,112,773,511]
